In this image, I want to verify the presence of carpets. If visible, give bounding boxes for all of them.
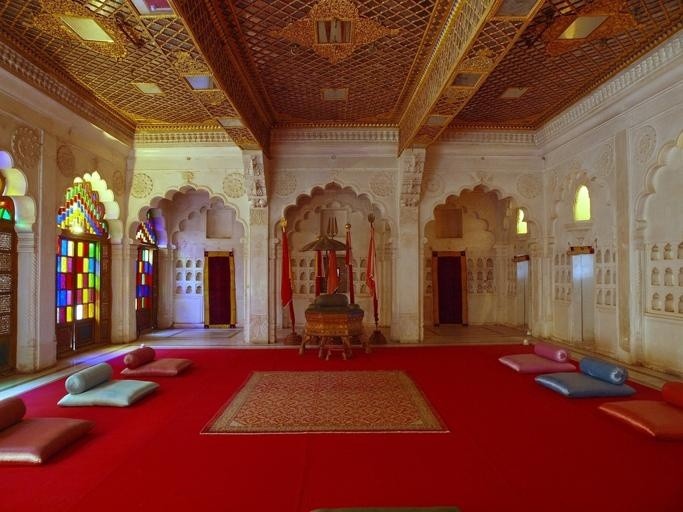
[200,367,452,438]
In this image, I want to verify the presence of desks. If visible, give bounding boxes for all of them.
[295,304,369,358]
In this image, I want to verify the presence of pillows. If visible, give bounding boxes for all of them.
[314,293,348,305]
[533,337,682,410]
[0,345,157,432]
